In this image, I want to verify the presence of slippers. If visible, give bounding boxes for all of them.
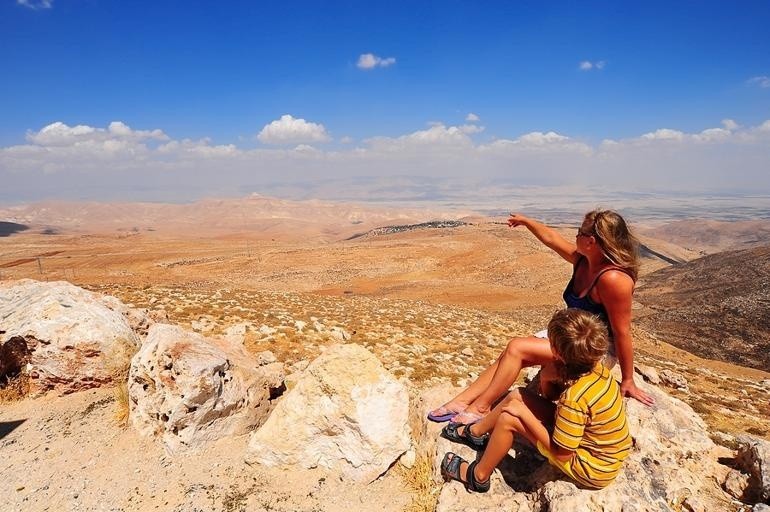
[428,407,463,422]
[450,412,485,424]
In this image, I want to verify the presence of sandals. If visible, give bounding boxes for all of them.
[442,422,489,451]
[442,452,490,494]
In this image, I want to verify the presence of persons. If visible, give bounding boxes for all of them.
[435,305,634,494]
[427,203,656,427]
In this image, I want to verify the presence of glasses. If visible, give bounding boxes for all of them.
[577,228,593,237]
